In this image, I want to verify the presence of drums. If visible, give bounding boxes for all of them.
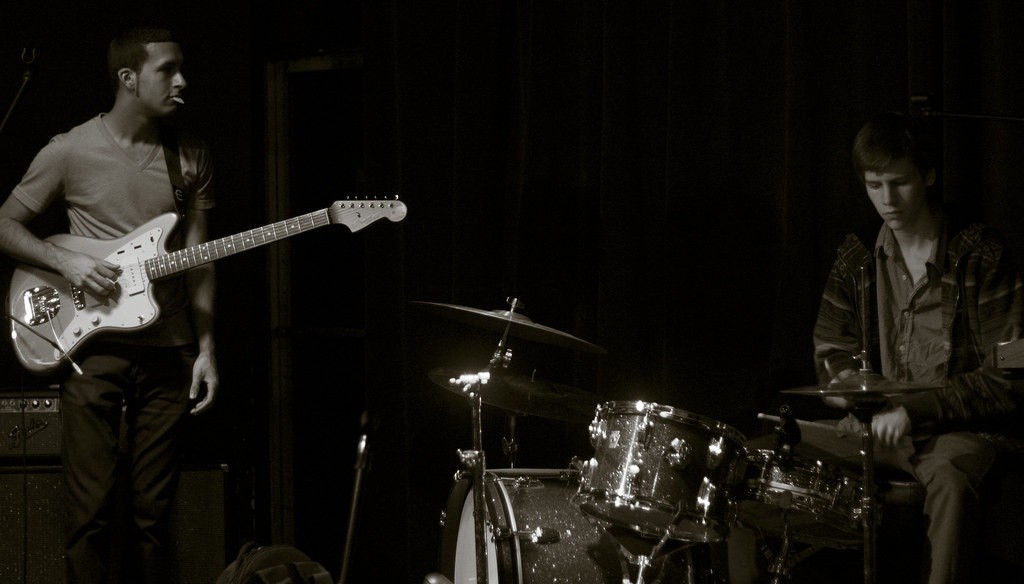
[736,446,873,548]
[577,399,748,544]
[438,470,683,584]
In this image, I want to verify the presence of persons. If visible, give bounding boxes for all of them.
[0,28,217,584]
[722,112,1022,584]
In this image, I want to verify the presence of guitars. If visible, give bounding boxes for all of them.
[11,194,409,376]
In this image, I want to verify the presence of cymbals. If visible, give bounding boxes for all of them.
[428,367,610,422]
[781,374,952,395]
[412,300,606,353]
